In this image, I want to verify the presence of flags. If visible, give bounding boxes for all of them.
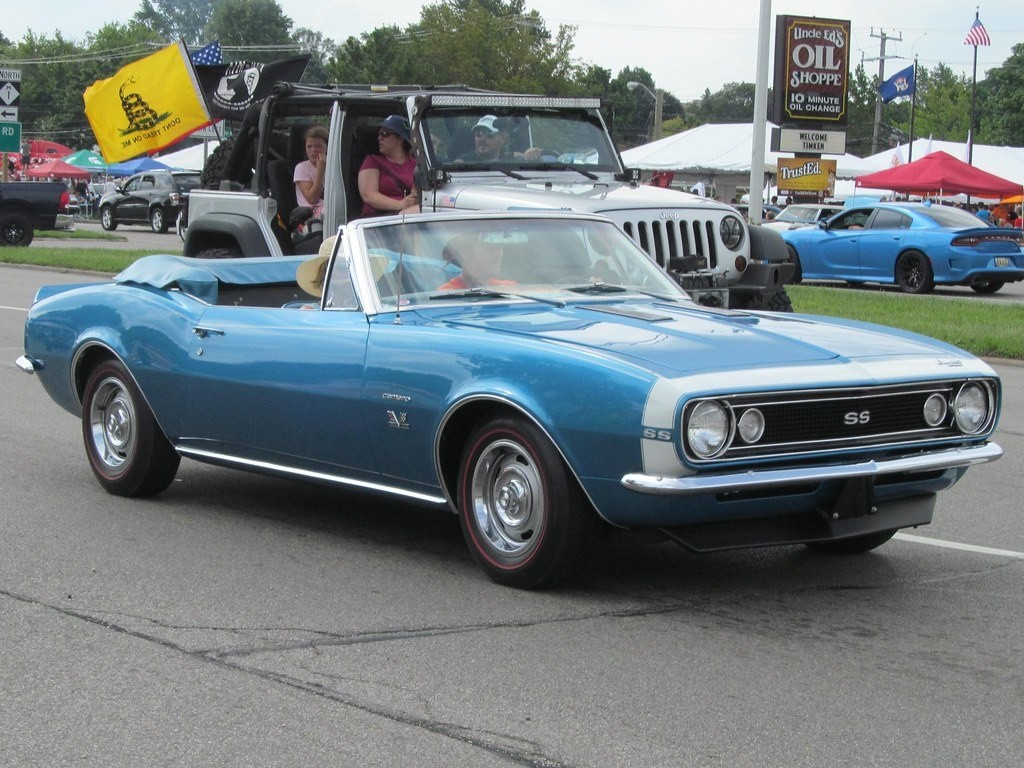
[83,39,212,163]
[878,64,917,104]
[964,130,971,164]
[190,40,224,65]
[925,135,932,157]
[964,18,991,46]
[888,143,905,169]
[194,52,313,123]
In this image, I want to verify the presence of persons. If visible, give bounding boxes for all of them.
[357,114,421,218]
[4,157,122,218]
[454,114,543,165]
[292,125,330,219]
[692,185,1024,233]
[295,234,388,310]
[436,228,519,290]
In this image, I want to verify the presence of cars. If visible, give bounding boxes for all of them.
[773,201,1024,295]
[729,194,885,232]
[96,168,209,234]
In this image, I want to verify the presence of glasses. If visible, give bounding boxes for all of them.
[476,129,496,139]
[378,130,398,138]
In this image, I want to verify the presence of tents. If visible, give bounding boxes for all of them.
[586,121,1024,223]
[23,139,221,179]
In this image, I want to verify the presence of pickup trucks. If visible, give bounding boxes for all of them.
[0,182,74,247]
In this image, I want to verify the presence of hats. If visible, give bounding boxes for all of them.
[471,115,500,133]
[294,234,389,299]
[442,226,502,268]
[375,115,412,150]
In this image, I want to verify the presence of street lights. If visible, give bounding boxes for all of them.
[626,80,663,142]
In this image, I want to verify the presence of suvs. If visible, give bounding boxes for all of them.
[217,52,792,310]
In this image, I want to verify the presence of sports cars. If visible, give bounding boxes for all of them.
[12,206,1005,593]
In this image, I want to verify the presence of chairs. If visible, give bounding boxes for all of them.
[349,124,381,224]
[268,123,319,226]
[445,127,476,162]
